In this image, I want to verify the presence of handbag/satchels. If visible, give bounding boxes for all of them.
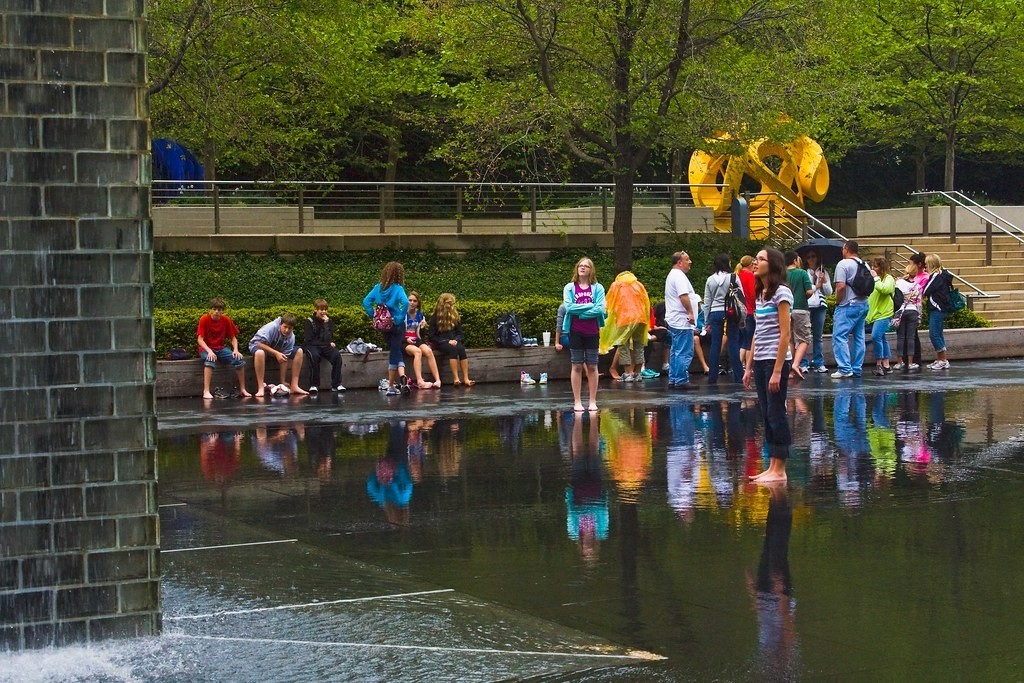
[370,302,395,333]
[166,346,192,360]
[889,316,901,329]
[949,283,965,310]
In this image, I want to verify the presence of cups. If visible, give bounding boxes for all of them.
[543,332,551,347]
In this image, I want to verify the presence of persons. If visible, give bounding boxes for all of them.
[562,257,606,410]
[196,298,253,399]
[363,261,475,395]
[249,314,309,397]
[555,239,953,389]
[302,299,346,392]
[741,249,792,483]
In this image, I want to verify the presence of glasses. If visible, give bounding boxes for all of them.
[577,264,591,268]
[806,255,816,259]
[752,256,769,263]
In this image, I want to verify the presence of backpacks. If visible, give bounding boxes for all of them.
[845,258,875,297]
[724,274,747,328]
[802,266,835,295]
[494,312,522,348]
[881,276,905,312]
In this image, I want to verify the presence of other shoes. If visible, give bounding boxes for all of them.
[668,383,699,389]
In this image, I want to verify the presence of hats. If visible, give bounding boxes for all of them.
[696,293,702,303]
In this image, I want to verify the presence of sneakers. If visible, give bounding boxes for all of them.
[347,339,366,355]
[831,372,861,378]
[718,364,829,375]
[893,362,905,370]
[522,337,538,347]
[214,383,346,399]
[520,371,536,385]
[908,363,919,369]
[927,361,950,369]
[539,372,548,383]
[617,363,669,382]
[378,375,416,396]
[872,365,893,376]
